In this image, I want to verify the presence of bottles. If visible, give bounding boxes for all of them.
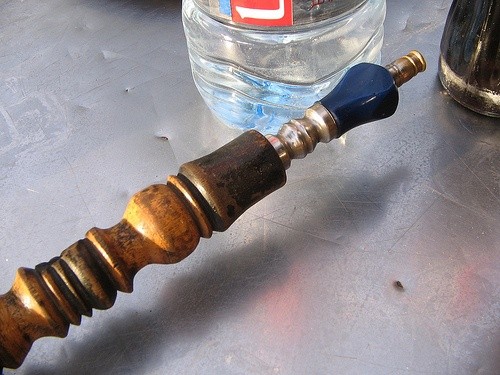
[438,0,500,119]
[180,0,390,136]
